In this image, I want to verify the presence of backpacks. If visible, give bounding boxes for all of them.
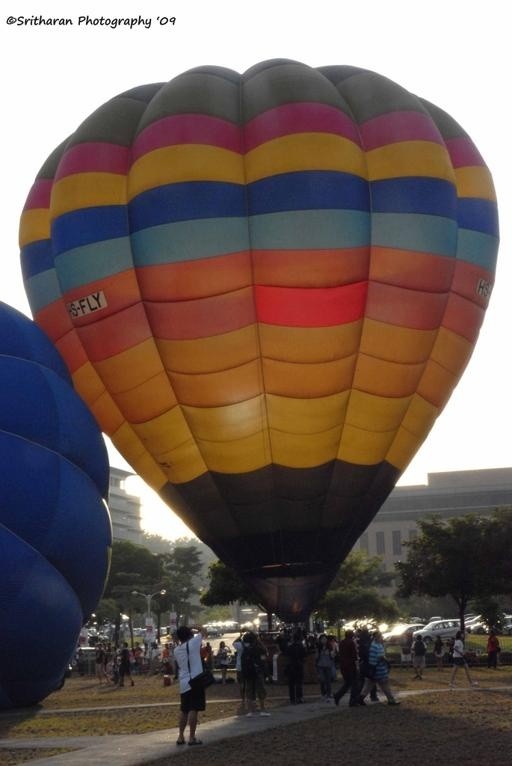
[239,640,261,677]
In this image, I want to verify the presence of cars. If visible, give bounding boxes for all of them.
[80,625,169,643]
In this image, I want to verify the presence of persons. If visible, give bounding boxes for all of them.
[93,628,500,745]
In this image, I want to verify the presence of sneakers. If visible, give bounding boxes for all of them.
[246,712,253,718]
[414,639,426,656]
[320,693,402,708]
[258,710,272,717]
[176,737,186,745]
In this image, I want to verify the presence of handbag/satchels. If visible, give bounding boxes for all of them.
[188,669,216,696]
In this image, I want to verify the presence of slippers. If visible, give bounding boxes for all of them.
[187,737,203,746]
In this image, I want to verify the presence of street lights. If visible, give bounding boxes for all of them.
[131,589,167,659]
